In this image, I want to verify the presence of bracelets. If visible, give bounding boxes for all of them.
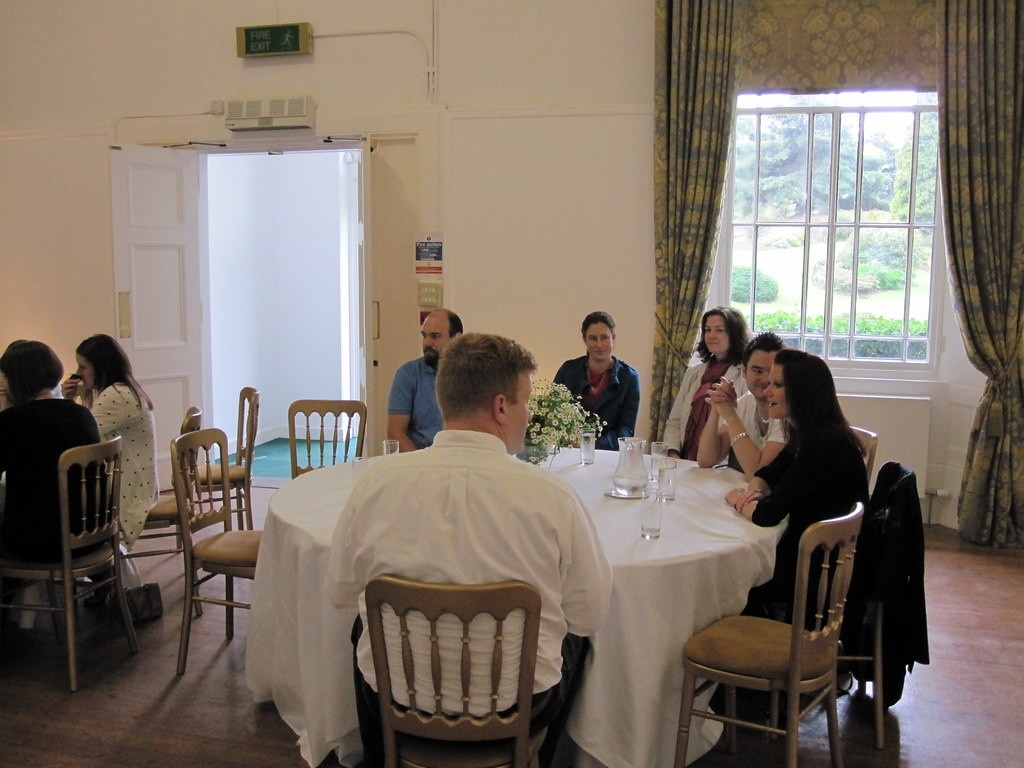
[731,433,748,447]
[755,489,763,493]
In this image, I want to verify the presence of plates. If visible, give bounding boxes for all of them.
[605,487,644,499]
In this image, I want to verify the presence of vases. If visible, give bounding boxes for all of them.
[516,446,551,469]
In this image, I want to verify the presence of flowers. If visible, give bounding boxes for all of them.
[515,380,607,464]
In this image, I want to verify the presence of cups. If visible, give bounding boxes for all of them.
[658,458,678,501]
[383,440,399,455]
[640,488,662,540]
[651,442,668,481]
[580,428,596,464]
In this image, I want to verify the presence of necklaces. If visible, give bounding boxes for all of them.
[757,405,768,424]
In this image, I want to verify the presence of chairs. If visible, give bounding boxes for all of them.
[0,435,141,692]
[758,460,919,752]
[172,428,265,675]
[288,398,367,484]
[675,501,865,767]
[117,406,203,617]
[849,425,877,481]
[364,574,548,768]
[192,386,262,530]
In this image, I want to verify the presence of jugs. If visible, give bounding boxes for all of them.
[613,437,648,497]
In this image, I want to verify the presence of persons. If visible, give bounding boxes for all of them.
[61,334,159,608]
[328,332,611,768]
[387,308,464,453]
[552,311,640,452]
[662,307,754,465]
[0,339,100,673]
[726,350,867,631]
[694,331,788,482]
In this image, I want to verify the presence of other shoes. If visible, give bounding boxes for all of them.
[84,585,110,607]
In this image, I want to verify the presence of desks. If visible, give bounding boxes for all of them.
[258,445,790,767]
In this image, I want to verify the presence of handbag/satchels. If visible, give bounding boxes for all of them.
[112,582,163,626]
